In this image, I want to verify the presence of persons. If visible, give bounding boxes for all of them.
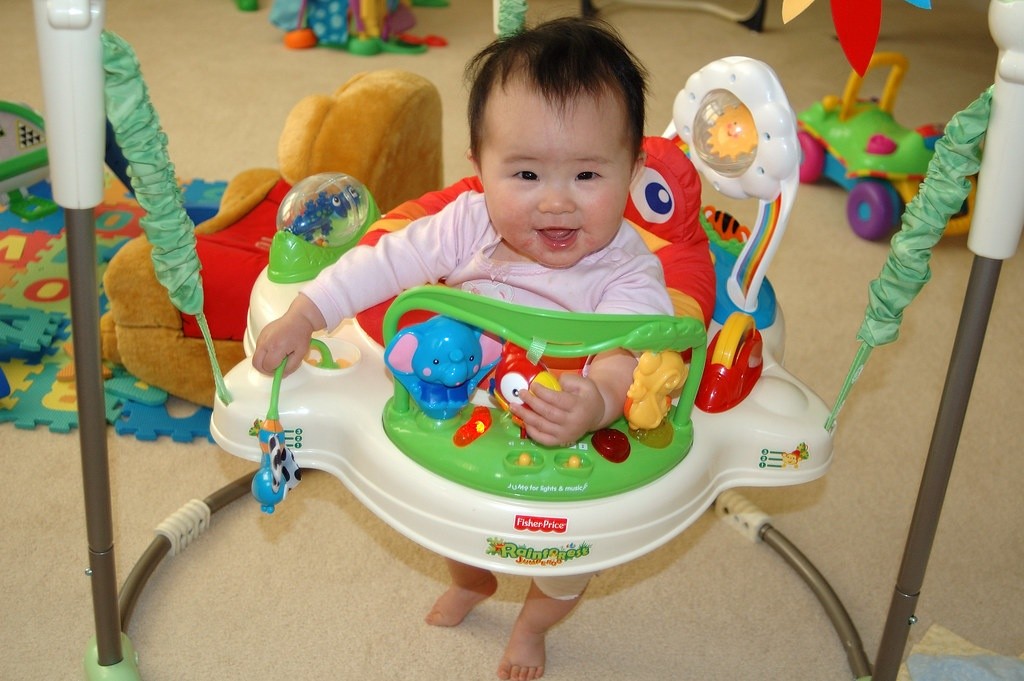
[252,17,675,681]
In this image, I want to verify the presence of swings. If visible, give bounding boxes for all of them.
[97,30,995,578]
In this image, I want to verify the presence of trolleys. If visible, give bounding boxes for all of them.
[796,51,977,238]
